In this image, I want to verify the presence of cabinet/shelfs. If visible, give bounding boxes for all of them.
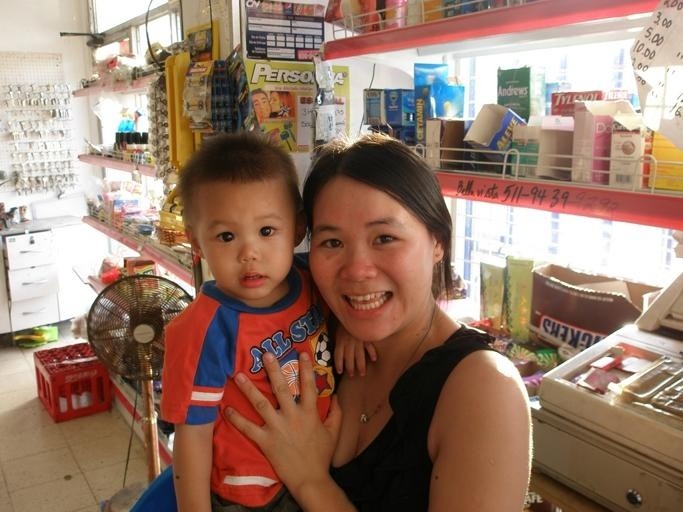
[0,213,113,337]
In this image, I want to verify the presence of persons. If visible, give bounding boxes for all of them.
[269,91,294,118]
[251,88,278,118]
[159,130,376,511]
[222,133,534,511]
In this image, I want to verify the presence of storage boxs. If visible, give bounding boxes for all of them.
[31,342,114,423]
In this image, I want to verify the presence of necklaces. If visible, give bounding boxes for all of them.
[358,301,435,423]
